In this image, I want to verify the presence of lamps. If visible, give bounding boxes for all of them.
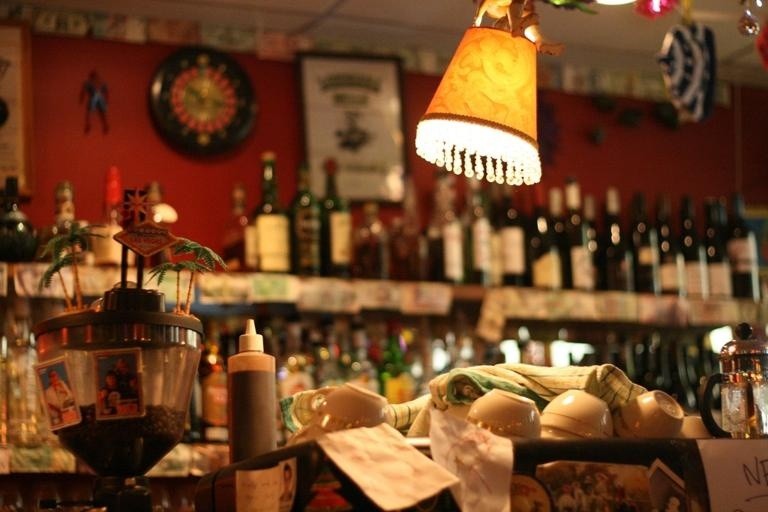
[414,0,543,187]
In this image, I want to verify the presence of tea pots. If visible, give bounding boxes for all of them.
[699,322,768,439]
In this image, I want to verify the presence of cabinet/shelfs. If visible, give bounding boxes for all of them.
[0,261,768,478]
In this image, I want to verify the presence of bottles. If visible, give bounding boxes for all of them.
[0,161,173,268]
[3,311,694,473]
[219,148,768,301]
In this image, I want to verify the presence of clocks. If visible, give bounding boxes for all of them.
[146,41,256,156]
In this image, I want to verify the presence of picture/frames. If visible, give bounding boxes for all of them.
[292,48,410,208]
[0,24,35,196]
